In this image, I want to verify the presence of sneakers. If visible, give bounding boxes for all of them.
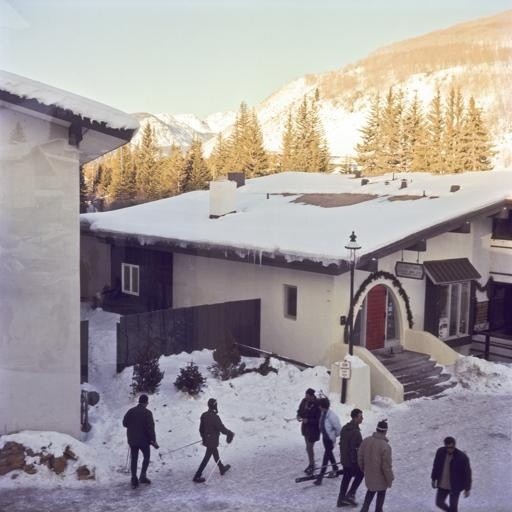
[219,463,231,475]
[131,476,151,488]
[332,465,339,471]
[336,493,359,508]
[312,479,322,486]
[192,476,206,483]
[303,464,315,474]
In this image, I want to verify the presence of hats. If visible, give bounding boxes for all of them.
[376,420,388,432]
[207,397,218,411]
[305,387,316,395]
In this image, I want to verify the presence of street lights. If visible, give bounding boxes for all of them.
[340,228,364,406]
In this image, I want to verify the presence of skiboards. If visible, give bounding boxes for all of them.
[295,462,343,483]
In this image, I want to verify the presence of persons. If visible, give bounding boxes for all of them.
[336,406,368,507]
[191,397,236,482]
[430,435,472,511]
[314,396,341,485]
[296,387,323,472]
[123,393,160,488]
[353,419,394,512]
